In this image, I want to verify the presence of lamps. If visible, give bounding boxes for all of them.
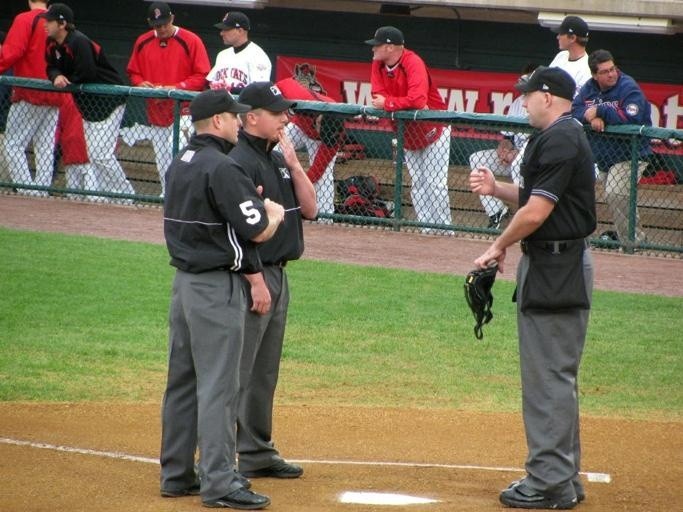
[537,11,679,36]
[151,0,269,11]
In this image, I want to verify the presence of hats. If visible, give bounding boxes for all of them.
[149,2,171,26]
[214,13,251,32]
[239,81,297,112]
[38,3,74,22]
[365,27,404,46]
[598,230,618,249]
[320,116,343,148]
[514,66,575,101]
[551,17,590,38]
[190,87,251,121]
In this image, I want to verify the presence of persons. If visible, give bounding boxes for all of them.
[464,64,542,232]
[225,78,321,488]
[470,65,598,509]
[363,27,457,238]
[0,0,62,199]
[467,16,594,234]
[154,86,285,509]
[125,3,213,200]
[270,79,345,224]
[199,12,272,120]
[36,4,138,206]
[54,87,121,203]
[570,50,653,248]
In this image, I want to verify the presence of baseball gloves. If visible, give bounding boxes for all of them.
[464,260,498,340]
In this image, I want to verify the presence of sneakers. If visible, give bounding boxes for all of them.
[499,480,583,510]
[241,460,303,477]
[202,475,271,509]
[488,207,509,230]
[160,488,201,496]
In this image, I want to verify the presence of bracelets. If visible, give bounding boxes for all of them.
[268,219,280,226]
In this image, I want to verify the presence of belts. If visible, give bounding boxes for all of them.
[520,240,571,252]
[264,258,286,268]
